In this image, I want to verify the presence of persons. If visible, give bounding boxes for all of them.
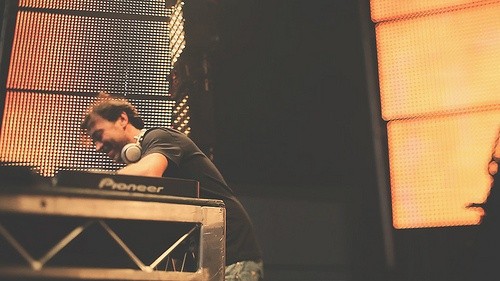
[79,90,264,281]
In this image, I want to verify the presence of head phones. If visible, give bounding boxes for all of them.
[121,128,148,165]
